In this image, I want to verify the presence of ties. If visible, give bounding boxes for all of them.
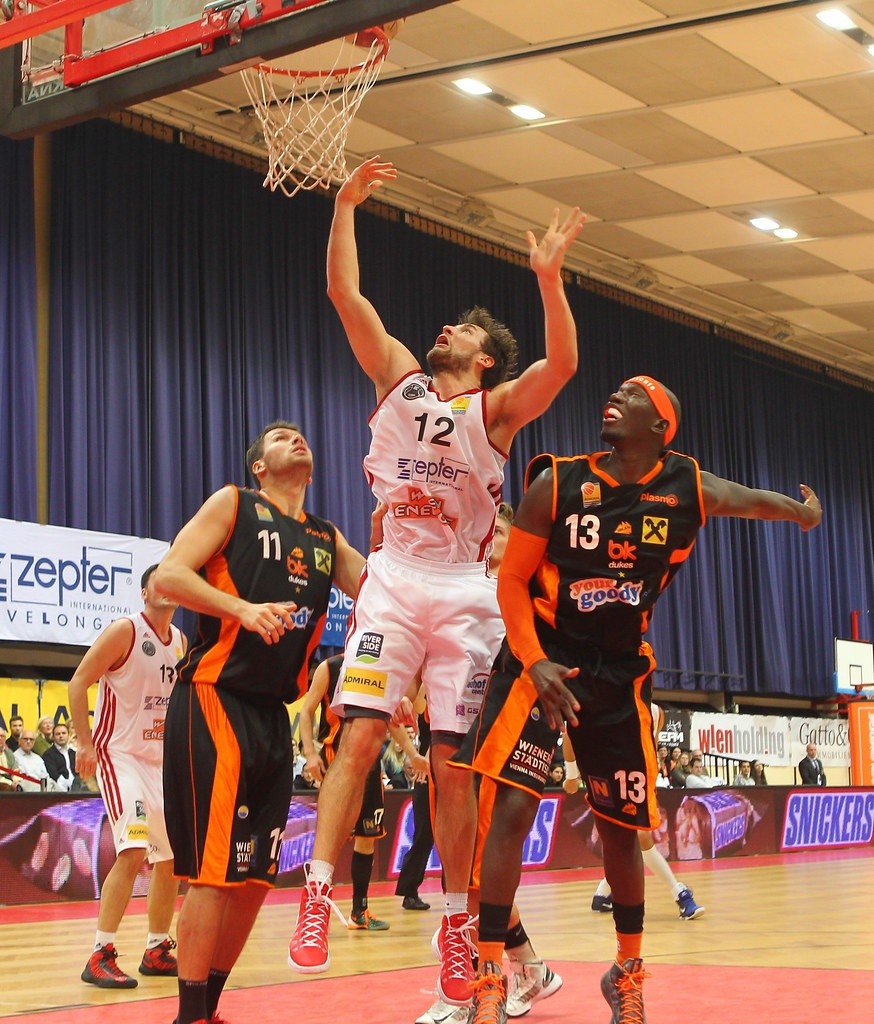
[813,759,817,770]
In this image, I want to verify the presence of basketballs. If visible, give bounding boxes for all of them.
[343,16,407,48]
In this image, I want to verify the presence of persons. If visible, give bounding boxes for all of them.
[67,564,179,988]
[153,419,367,1024]
[0,716,78,792]
[292,739,319,790]
[656,742,827,788]
[288,153,822,1024]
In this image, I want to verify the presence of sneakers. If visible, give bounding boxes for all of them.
[601,958,653,1024]
[80,944,138,989]
[139,936,178,977]
[591,894,614,913]
[415,997,474,1024]
[675,888,705,920]
[431,912,479,1006]
[472,961,507,1024]
[505,961,562,1017]
[287,862,348,973]
[401,896,430,910]
[348,908,389,930]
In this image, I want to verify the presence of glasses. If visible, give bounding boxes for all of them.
[19,737,35,741]
